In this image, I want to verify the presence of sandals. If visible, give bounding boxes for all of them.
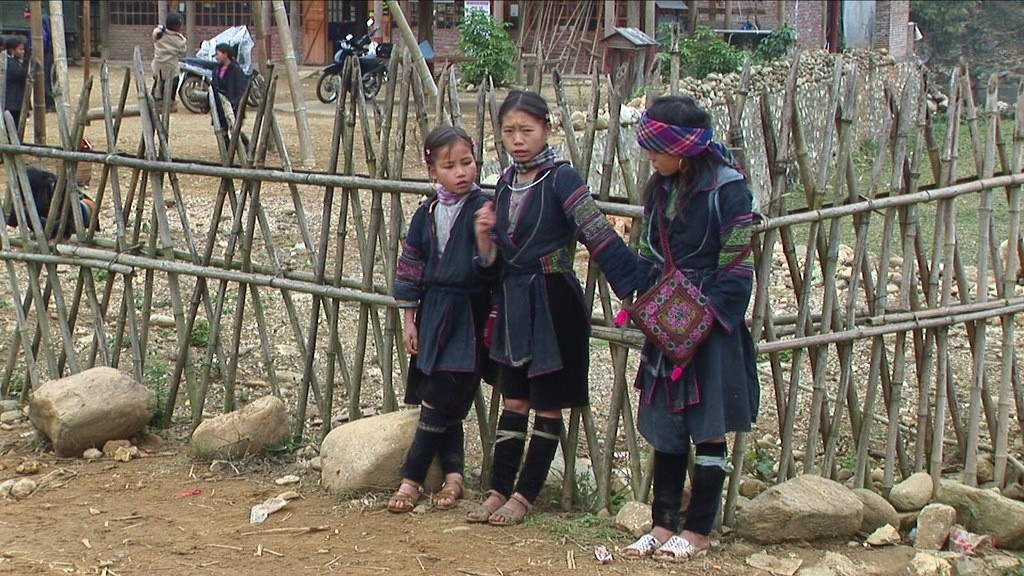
[488,495,532,525]
[435,480,464,510]
[466,490,506,521]
[387,480,424,512]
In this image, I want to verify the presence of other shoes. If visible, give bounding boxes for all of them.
[46,106,56,112]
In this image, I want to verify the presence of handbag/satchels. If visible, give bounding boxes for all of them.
[615,270,714,381]
[484,305,498,348]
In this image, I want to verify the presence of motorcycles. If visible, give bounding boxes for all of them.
[177,25,266,114]
[315,17,393,104]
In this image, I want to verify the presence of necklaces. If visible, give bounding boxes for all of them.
[507,169,551,191]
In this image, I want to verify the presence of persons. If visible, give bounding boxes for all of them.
[150,14,187,100]
[387,125,499,513]
[465,90,637,524]
[24,4,56,113]
[207,43,250,151]
[5,35,32,142]
[622,96,761,563]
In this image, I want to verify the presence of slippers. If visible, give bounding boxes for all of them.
[651,535,708,561]
[620,534,663,559]
